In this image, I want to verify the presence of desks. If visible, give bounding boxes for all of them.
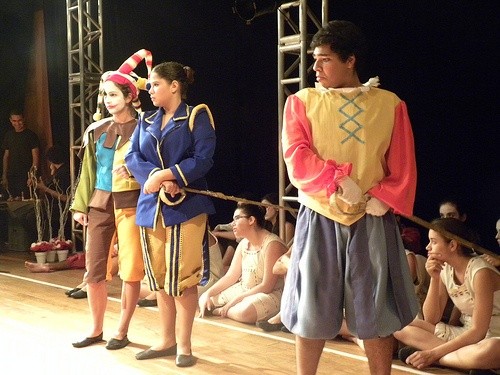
[0,198,46,252]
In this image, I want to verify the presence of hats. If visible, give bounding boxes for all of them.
[92,49,152,122]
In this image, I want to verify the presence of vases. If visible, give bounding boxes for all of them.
[35,251,47,263]
[57,250,69,262]
[47,250,56,262]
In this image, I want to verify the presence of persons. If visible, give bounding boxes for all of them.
[64,192,500,375]
[280,20,419,375]
[124,62,218,367]
[69,49,153,350]
[30,144,78,246]
[0,111,40,244]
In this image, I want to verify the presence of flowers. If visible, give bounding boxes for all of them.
[30,238,72,252]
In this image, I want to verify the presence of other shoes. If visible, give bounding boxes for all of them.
[72,332,104,348]
[398,346,418,364]
[138,298,158,307]
[470,369,489,375]
[135,343,178,359]
[176,350,193,366]
[71,290,87,298]
[105,335,129,349]
[198,305,219,316]
[256,319,291,332]
[65,287,80,295]
